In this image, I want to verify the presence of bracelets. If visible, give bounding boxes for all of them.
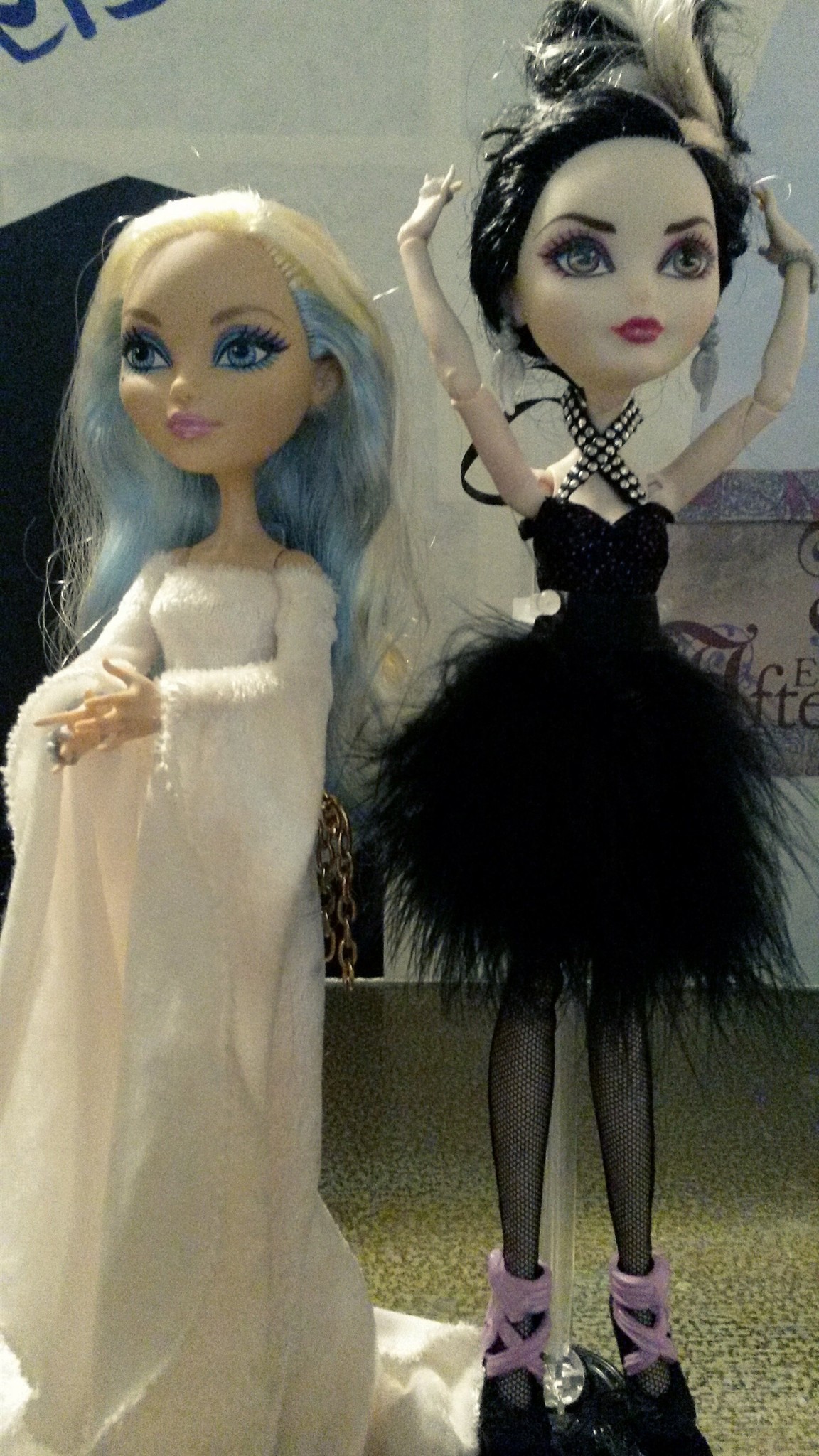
[779,248,819,294]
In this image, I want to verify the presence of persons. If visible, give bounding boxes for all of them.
[361,0,819,1456]
[0,187,488,1456]
